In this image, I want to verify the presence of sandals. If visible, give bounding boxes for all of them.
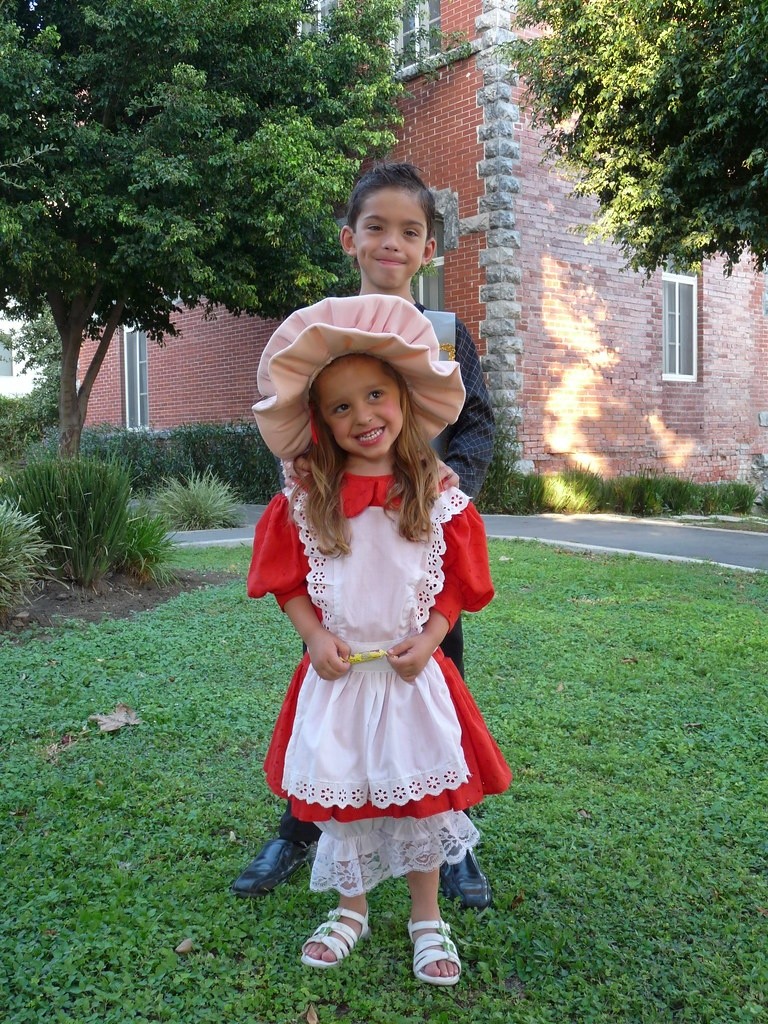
[302,906,369,969]
[407,917,461,984]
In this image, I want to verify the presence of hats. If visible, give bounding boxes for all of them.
[252,295,468,459]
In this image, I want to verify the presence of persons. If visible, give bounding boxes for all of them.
[230,161,519,988]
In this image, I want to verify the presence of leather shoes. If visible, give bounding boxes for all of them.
[233,837,310,899]
[440,827,491,907]
